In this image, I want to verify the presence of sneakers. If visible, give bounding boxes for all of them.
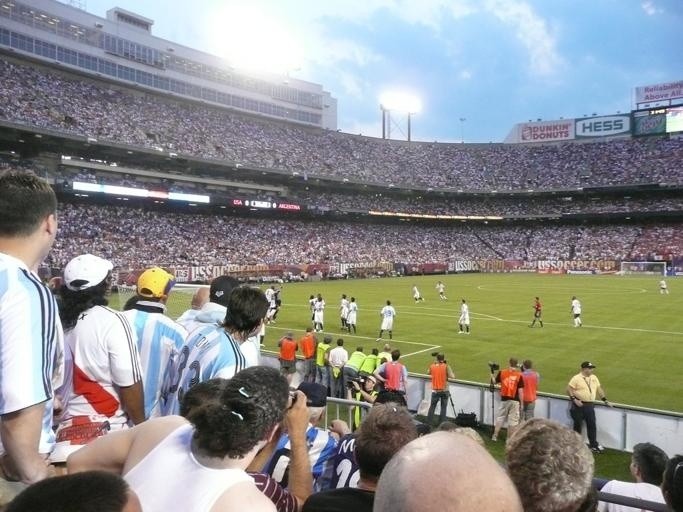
[592,445,604,453]
[492,433,498,441]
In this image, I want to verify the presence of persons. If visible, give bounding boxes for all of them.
[1,46,681,280]
[52,254,416,512]
[415,347,681,512]
[660,277,668,294]
[529,296,542,328]
[568,296,582,328]
[436,281,446,299]
[8,469,144,512]
[372,430,525,507]
[458,300,471,337]
[0,167,63,512]
[412,285,422,304]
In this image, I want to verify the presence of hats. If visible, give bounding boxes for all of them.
[137,267,176,298]
[64,253,113,291]
[210,275,240,305]
[366,375,376,383]
[294,381,327,407]
[581,362,596,368]
